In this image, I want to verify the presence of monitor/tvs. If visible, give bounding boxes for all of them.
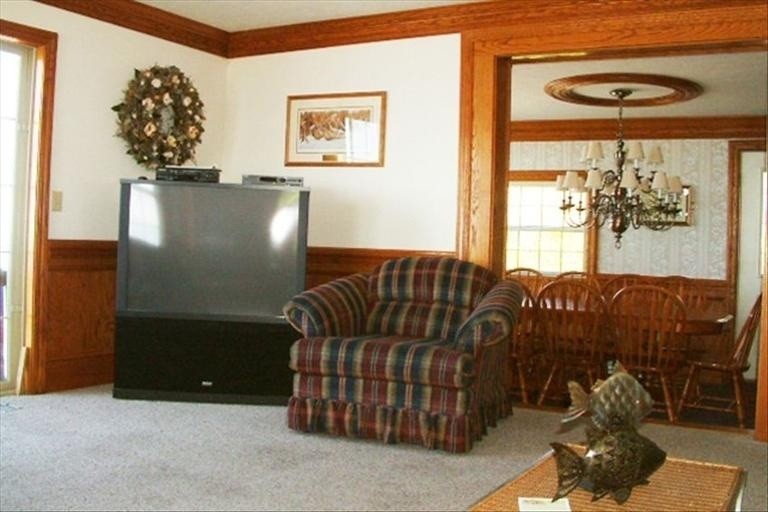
[114,178,312,324]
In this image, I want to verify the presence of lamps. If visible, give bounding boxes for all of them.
[555,88,682,249]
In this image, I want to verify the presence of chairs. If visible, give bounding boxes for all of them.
[502,278,536,407]
[654,275,700,292]
[675,292,762,429]
[555,271,602,295]
[702,280,727,297]
[536,280,607,407]
[505,268,546,298]
[608,284,687,423]
[602,275,653,297]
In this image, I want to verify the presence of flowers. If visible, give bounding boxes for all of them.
[110,61,206,171]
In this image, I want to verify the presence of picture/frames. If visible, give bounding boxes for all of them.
[637,183,693,228]
[284,90,387,168]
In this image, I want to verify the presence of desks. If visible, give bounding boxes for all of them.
[467,442,748,512]
[519,297,735,334]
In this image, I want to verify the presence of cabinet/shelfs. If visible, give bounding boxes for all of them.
[112,318,302,407]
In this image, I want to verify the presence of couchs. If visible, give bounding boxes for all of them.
[281,255,522,452]
